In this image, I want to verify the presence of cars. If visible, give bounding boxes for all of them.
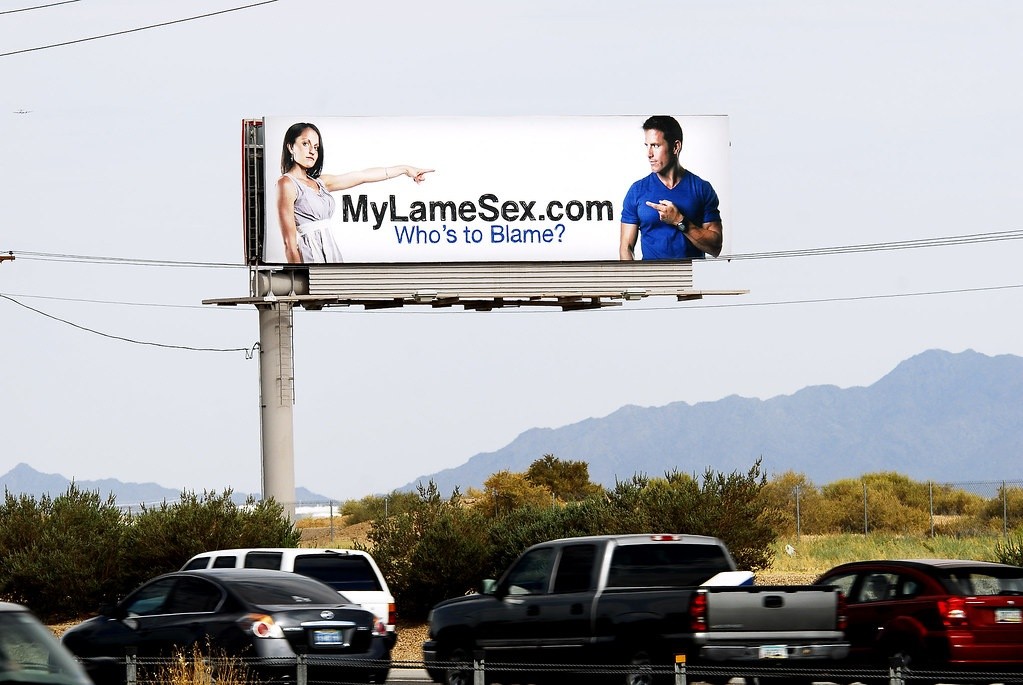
[810,559,1023,685]
[0,570,398,685]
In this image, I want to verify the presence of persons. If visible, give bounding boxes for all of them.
[619,115,723,261]
[277,124,435,264]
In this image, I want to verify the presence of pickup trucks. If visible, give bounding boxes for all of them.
[422,534,851,685]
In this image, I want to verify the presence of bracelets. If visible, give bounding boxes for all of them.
[385,167,391,180]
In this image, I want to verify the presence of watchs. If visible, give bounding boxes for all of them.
[677,216,689,233]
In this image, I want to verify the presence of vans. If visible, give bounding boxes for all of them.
[130,548,397,650]
